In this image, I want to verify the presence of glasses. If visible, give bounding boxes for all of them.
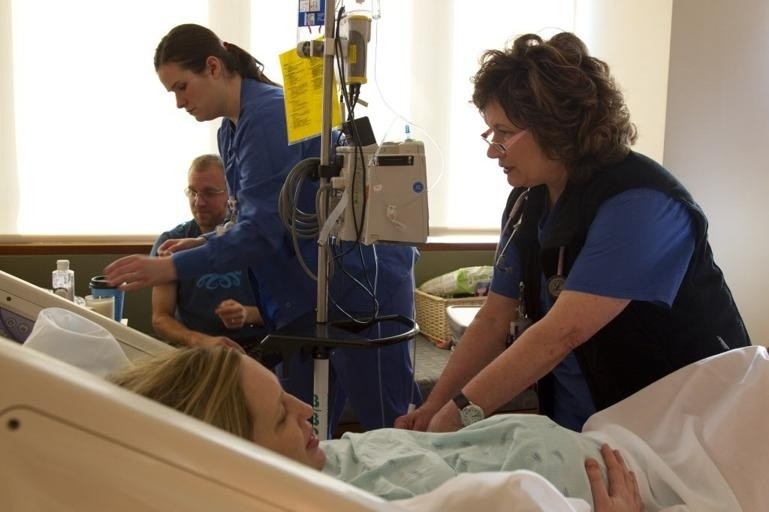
[182,186,228,200]
[480,126,529,155]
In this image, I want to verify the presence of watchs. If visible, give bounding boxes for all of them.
[451,391,485,427]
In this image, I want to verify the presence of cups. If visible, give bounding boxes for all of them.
[89,274,127,322]
[85,295,115,321]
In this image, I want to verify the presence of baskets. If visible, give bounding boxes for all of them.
[413,287,489,349]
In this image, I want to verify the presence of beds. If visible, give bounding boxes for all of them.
[1,270,769,512]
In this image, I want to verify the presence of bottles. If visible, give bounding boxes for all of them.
[52,259,75,302]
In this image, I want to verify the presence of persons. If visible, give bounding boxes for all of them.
[151,155,263,354]
[395,33,751,432]
[105,344,769,512]
[103,24,421,441]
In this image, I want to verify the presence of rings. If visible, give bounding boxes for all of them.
[232,319,234,324]
